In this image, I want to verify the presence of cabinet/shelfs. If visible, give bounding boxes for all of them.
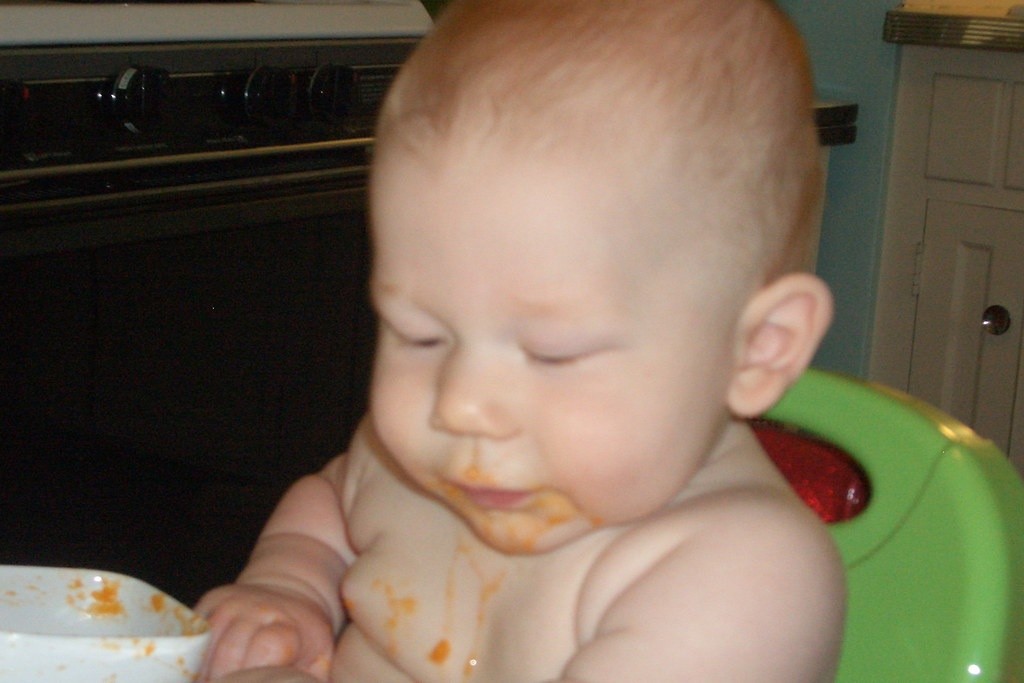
[863,43,1024,482]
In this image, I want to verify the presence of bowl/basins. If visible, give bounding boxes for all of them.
[0,561,212,683]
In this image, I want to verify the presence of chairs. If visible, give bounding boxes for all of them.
[750,366,1024,683]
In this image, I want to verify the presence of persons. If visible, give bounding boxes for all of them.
[192,0,850,683]
